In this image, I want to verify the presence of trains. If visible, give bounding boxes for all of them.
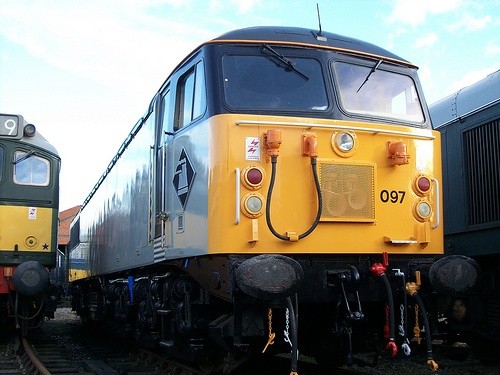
[0,110,61,333]
[57,0,474,375]
[425,67,500,374]
